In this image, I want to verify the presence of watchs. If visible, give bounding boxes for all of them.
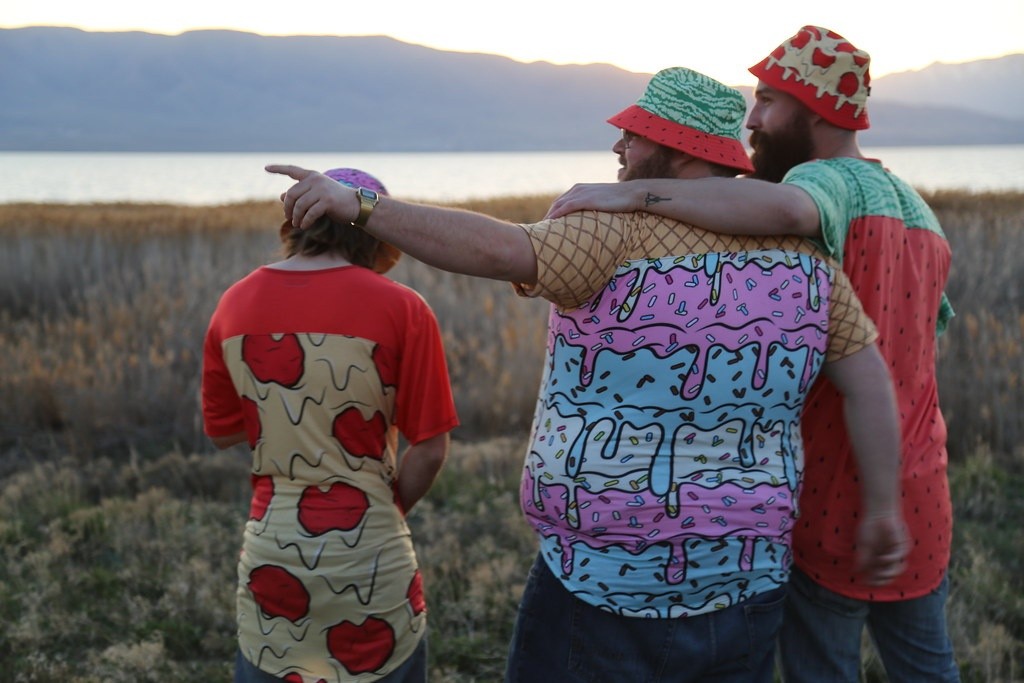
[351,188,379,230]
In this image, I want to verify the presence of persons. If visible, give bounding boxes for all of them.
[199,167,459,683]
[544,24,963,683]
[260,66,910,683]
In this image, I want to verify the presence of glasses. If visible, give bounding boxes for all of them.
[621,128,640,148]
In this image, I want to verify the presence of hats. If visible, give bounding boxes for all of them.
[748,25,872,130]
[323,168,402,274]
[605,67,755,174]
